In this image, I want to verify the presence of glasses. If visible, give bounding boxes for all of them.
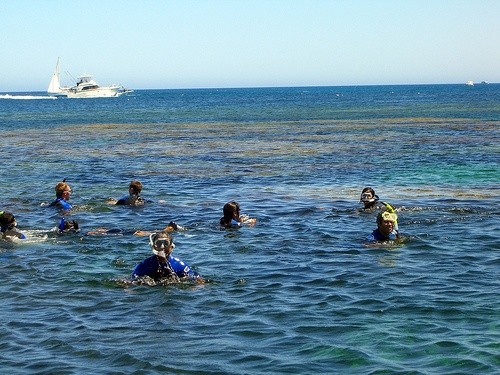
[14,223,18,228]
[382,211,397,221]
[169,221,177,231]
[361,193,372,199]
[153,238,172,248]
[63,189,73,194]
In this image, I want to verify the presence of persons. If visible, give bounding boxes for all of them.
[368,211,400,241]
[0,212,26,243]
[49,218,186,238]
[361,188,394,211]
[50,181,73,210]
[220,200,255,228]
[130,233,204,286]
[115,179,166,206]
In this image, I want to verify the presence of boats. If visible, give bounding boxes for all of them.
[49,66,127,98]
[466,80,474,86]
